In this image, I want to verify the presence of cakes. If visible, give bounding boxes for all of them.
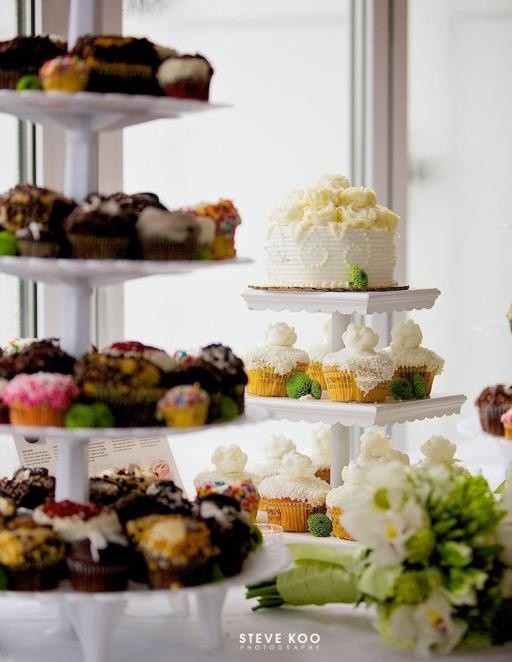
[262,175,401,290]
[0,429,457,591]
[498,410,512,441]
[474,384,512,435]
[247,319,442,400]
[0,33,214,100]
[0,337,247,427]
[1,183,239,260]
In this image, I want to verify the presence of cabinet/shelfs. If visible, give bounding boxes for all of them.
[241,285,467,551]
[0,88,293,661]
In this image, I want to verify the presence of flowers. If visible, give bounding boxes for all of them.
[243,435,512,662]
